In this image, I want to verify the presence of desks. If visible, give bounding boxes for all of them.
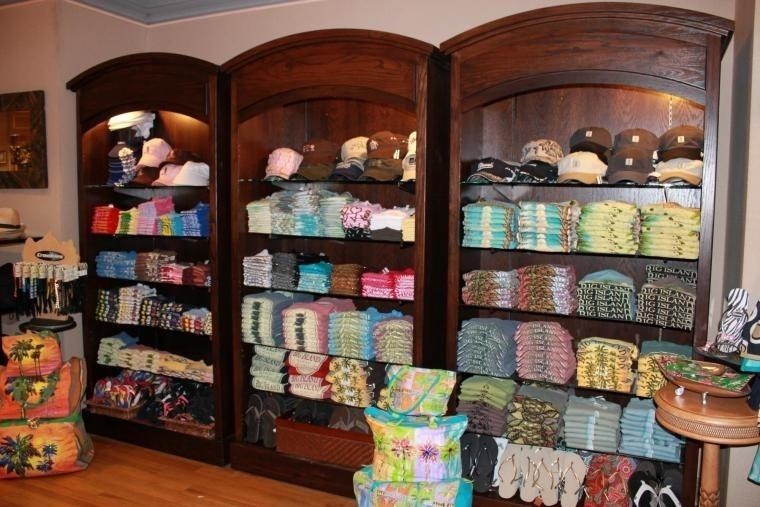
[1,233,43,247]
[653,379,760,507]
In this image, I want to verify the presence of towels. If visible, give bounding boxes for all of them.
[634,341,693,400]
[511,316,584,384]
[639,203,699,260]
[240,189,415,411]
[517,265,580,314]
[457,317,526,377]
[636,263,698,333]
[505,381,575,449]
[461,202,520,250]
[461,268,518,308]
[456,373,521,436]
[562,394,621,456]
[577,270,639,318]
[620,396,688,464]
[90,195,214,385]
[578,199,639,255]
[517,199,582,251]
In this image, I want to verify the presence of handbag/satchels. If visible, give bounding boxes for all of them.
[353,365,473,507]
[0,331,95,480]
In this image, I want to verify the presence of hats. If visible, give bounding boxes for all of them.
[264,132,417,183]
[131,139,211,187]
[0,208,26,233]
[465,125,703,187]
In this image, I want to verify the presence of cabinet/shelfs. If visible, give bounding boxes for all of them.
[222,27,447,498]
[65,52,233,467]
[441,0,737,507]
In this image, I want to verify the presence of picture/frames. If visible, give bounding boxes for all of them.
[0,89,49,189]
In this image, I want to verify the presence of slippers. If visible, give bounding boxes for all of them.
[718,288,750,353]
[474,434,682,507]
[243,393,371,448]
